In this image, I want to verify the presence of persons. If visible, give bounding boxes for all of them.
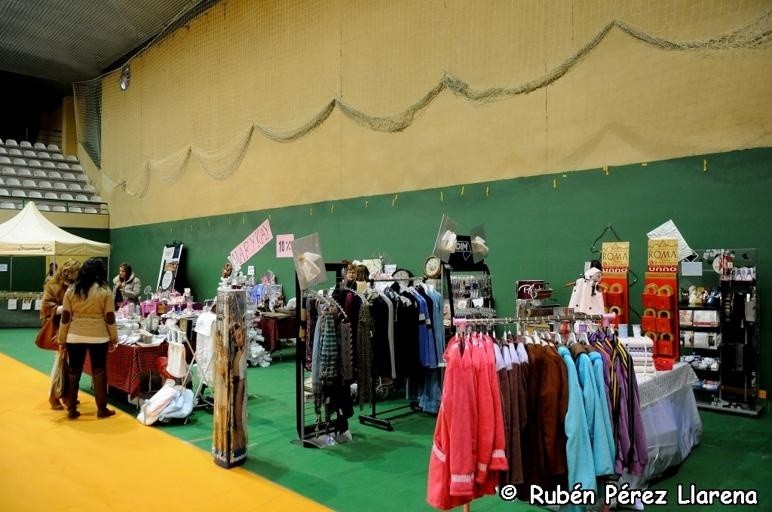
[355,264,370,296]
[347,263,357,290]
[58,255,119,421]
[44,263,57,283]
[39,259,83,411]
[231,323,247,457]
[112,262,142,306]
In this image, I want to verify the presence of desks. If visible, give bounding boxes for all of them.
[83,341,195,396]
[634,362,703,481]
[258,311,297,354]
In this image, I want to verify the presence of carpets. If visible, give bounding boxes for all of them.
[1,325,772,512]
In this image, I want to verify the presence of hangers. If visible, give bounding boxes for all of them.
[455,314,616,346]
[304,276,433,317]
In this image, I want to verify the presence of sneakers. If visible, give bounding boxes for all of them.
[49,397,80,410]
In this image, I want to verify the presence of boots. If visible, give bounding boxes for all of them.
[94,393,116,417]
[66,395,80,418]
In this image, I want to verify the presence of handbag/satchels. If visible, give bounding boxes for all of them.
[53,358,67,399]
[35,317,61,350]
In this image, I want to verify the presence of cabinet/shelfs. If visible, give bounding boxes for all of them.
[677,247,766,417]
